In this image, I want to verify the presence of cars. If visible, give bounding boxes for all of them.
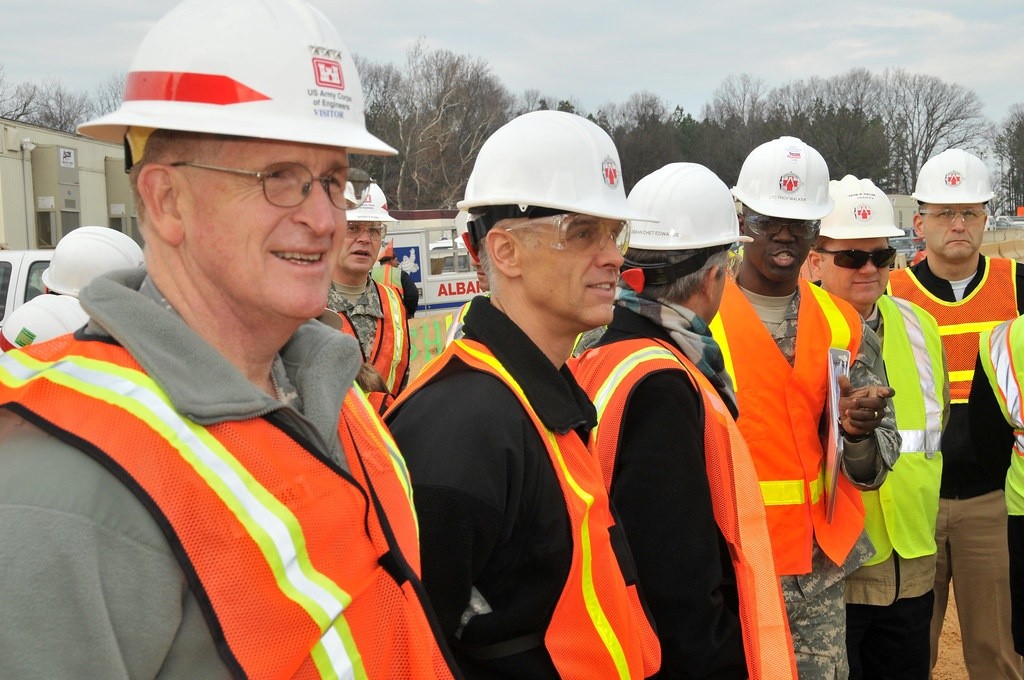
[0,249,55,330]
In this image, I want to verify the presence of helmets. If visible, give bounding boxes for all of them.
[41,225,147,300]
[817,174,905,240]
[0,292,91,354]
[74,3,398,155]
[616,161,754,250]
[910,148,994,203]
[343,176,399,222]
[732,135,835,220]
[457,110,660,223]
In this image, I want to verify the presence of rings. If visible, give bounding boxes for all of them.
[875,412,878,419]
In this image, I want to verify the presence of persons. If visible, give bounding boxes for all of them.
[0,226,145,352]
[383,110,663,680]
[584,136,1024,680]
[0,0,463,680]
[325,171,419,400]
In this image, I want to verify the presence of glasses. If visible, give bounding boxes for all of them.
[169,151,376,211]
[504,208,630,257]
[344,222,386,241]
[919,207,988,225]
[816,245,896,269]
[741,205,821,239]
[724,248,743,281]
